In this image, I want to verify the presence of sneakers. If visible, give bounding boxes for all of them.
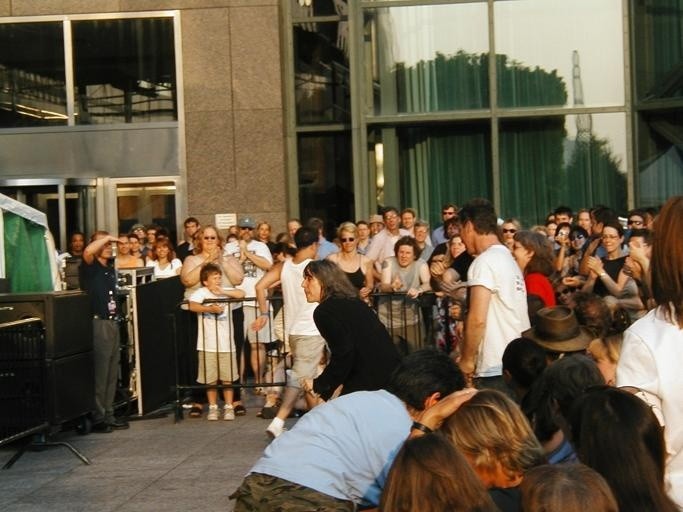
[207,405,220,421]
[223,404,235,421]
[266,416,284,439]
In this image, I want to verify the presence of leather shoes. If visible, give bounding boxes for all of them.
[108,420,128,429]
[92,423,113,433]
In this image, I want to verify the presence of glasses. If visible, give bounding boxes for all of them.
[627,220,644,225]
[558,232,569,237]
[570,233,584,240]
[503,228,516,233]
[443,212,455,215]
[341,238,354,242]
[601,234,619,239]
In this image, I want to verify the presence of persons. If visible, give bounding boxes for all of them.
[59,199,681,510]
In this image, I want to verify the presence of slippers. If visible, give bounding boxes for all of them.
[189,405,203,416]
[234,401,246,415]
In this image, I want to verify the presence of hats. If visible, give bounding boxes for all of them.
[521,304,594,353]
[131,223,145,230]
[368,214,383,224]
[237,216,255,228]
